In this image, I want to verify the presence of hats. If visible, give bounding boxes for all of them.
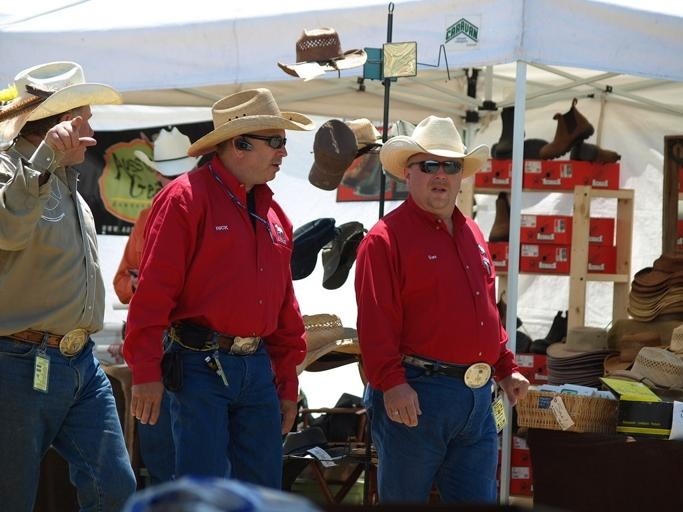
[604,331,669,372]
[297,314,361,376]
[628,250,683,322]
[344,118,382,150]
[322,221,367,290]
[664,325,683,361]
[283,427,352,468]
[547,326,612,386]
[277,27,367,81]
[0,62,123,152]
[134,128,203,176]
[291,217,336,281]
[380,115,490,179]
[188,89,314,158]
[309,119,358,190]
[609,347,683,392]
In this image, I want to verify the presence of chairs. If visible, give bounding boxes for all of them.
[279,348,382,512]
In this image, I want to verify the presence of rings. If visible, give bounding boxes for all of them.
[391,411,398,416]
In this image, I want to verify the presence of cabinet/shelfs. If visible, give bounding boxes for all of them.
[457,178,636,512]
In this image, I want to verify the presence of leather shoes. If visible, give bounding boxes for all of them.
[495,106,525,160]
[491,139,548,162]
[570,143,622,163]
[530,309,568,355]
[489,192,510,242]
[539,98,595,160]
[516,316,532,354]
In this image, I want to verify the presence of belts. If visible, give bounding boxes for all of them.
[400,354,495,389]
[169,323,263,356]
[11,329,92,356]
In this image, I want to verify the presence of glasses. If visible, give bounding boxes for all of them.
[407,160,463,174]
[160,174,178,180]
[240,133,287,148]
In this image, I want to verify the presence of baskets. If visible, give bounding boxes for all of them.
[518,390,620,434]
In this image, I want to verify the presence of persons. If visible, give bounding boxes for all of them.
[114,127,203,487]
[354,116,530,508]
[124,89,316,491]
[1,62,138,512]
